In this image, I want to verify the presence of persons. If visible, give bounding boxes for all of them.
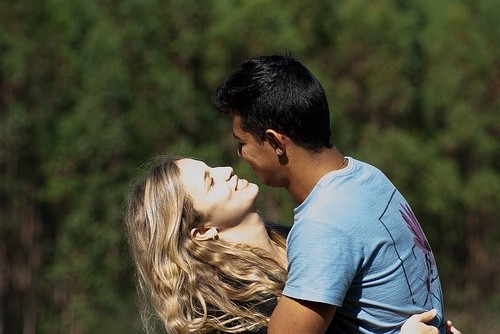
[211,54,445,334]
[119,157,463,334]
